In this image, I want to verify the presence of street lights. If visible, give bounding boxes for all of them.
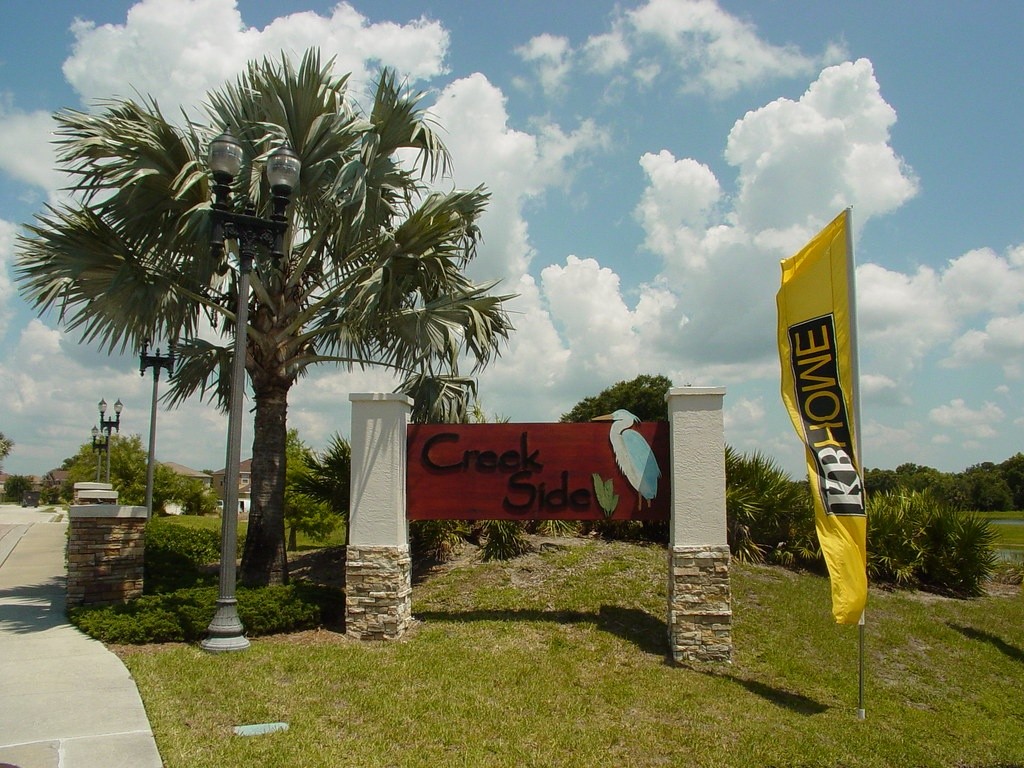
[89,425,108,481]
[138,325,178,523]
[97,396,125,481]
[196,122,304,655]
